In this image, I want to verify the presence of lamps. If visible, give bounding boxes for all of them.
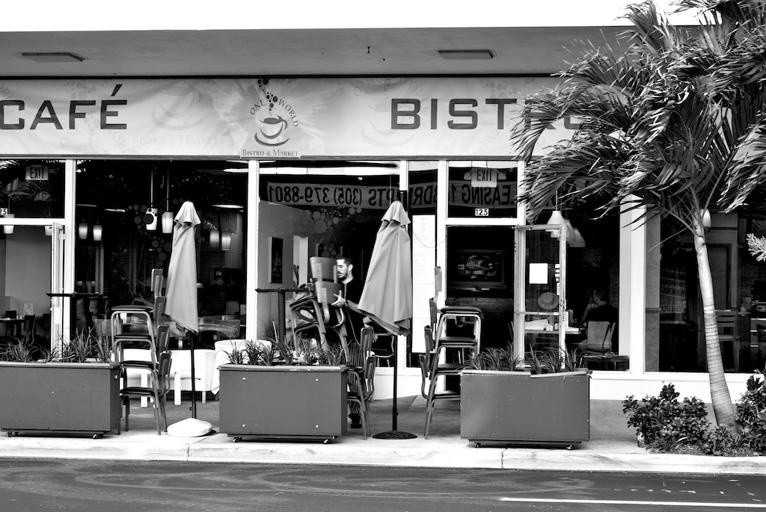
[77,208,101,243]
[210,228,233,251]
[2,190,15,234]
[548,175,588,249]
[144,156,173,235]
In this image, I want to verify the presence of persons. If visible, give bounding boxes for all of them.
[532,292,559,325]
[570,282,619,369]
[325,254,369,430]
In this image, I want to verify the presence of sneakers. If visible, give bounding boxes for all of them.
[350,417,362,428]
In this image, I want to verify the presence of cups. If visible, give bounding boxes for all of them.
[158,80,203,118]
[254,106,288,139]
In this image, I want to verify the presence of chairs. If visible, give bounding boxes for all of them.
[700,311,766,371]
[110,268,175,434]
[4,310,36,350]
[289,253,374,440]
[574,319,614,367]
[419,265,486,441]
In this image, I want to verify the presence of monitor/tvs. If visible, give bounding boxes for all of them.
[527,261,553,286]
[447,249,504,288]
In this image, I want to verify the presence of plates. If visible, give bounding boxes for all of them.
[153,106,206,129]
[254,130,291,146]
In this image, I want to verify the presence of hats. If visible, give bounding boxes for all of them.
[538,292,559,310]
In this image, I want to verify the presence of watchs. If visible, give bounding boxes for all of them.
[345,298,350,310]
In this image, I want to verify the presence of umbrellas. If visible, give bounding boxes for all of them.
[160,201,203,419]
[355,198,414,432]
